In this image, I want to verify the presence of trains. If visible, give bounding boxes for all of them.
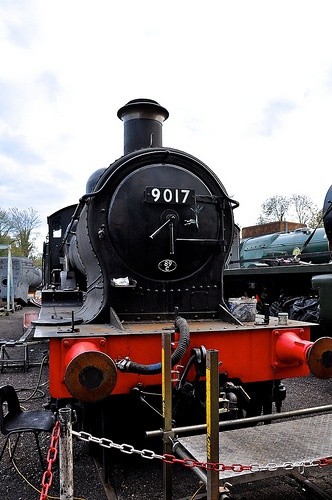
[30,97,331,484]
[222,222,331,312]
